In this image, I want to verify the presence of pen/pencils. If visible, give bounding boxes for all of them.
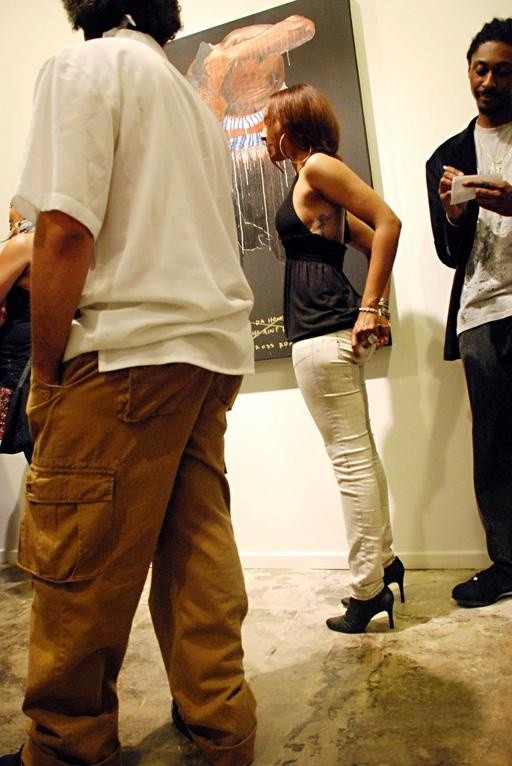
[443,165,464,175]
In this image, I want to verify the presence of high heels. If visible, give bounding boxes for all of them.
[326,556,405,634]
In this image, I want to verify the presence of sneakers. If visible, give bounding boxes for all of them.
[452,562,512,606]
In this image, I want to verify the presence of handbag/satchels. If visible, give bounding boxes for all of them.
[0,386,18,451]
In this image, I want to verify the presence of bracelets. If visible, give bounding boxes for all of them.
[356,306,379,313]
[377,296,390,319]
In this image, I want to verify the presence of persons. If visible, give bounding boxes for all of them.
[2,196,45,467]
[0,0,256,764]
[260,83,407,635]
[423,17,510,610]
